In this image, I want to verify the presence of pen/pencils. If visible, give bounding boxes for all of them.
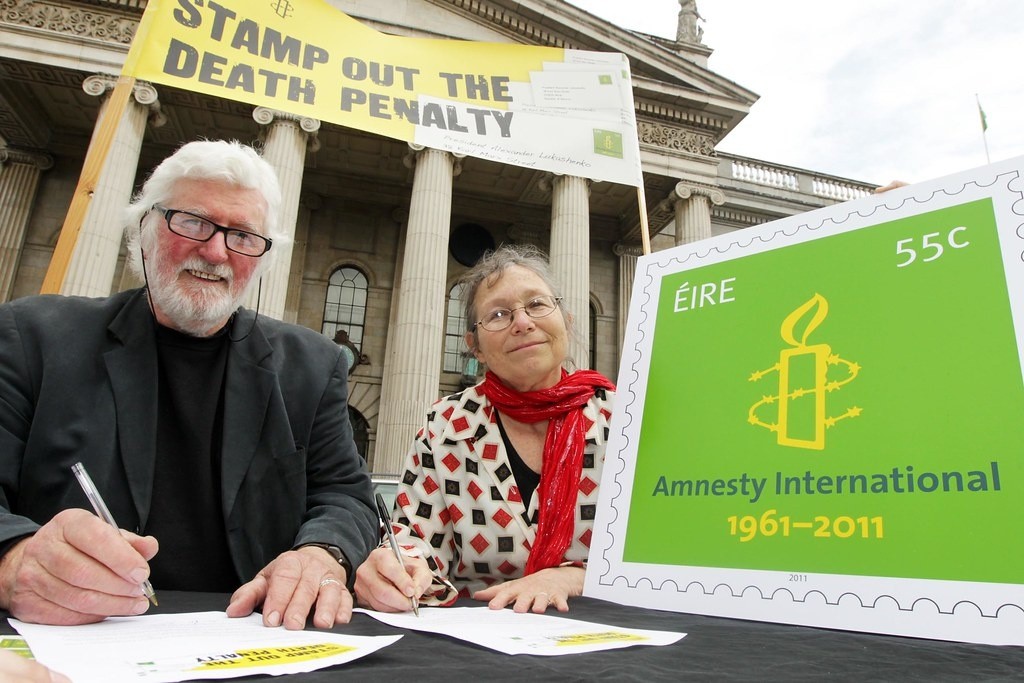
[72,463,161,608]
[374,492,420,618]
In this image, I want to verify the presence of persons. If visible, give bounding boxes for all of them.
[353,244,616,613]
[0,140,380,630]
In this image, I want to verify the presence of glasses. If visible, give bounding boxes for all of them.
[473,295,563,331]
[153,203,273,257]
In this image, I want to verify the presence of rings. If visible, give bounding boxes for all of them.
[537,592,550,598]
[318,578,340,593]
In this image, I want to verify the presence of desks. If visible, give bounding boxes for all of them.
[0,596,1024,683]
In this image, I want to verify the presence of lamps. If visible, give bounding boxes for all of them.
[460,351,478,385]
